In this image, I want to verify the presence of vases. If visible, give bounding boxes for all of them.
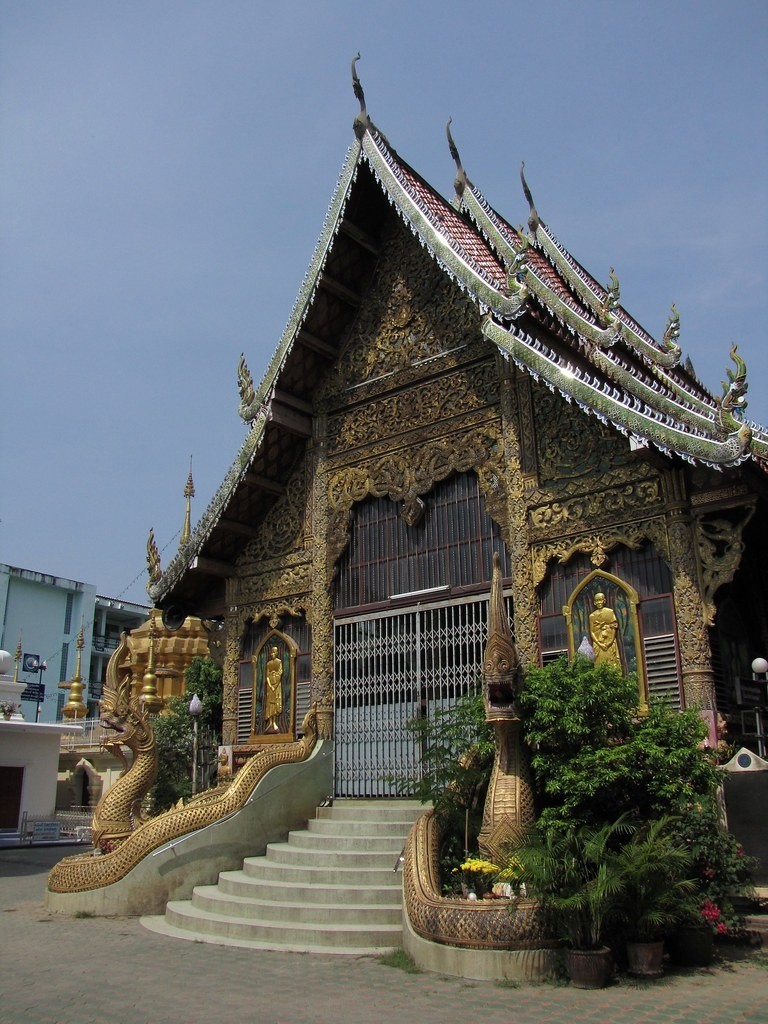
[672,923,706,967]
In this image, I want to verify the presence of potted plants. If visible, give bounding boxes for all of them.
[518,814,692,988]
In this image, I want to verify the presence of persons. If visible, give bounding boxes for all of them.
[218,748,231,778]
[263,646,283,731]
[589,592,623,676]
[200,619,226,661]
[699,505,756,601]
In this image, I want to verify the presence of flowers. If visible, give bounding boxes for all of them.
[680,900,726,937]
